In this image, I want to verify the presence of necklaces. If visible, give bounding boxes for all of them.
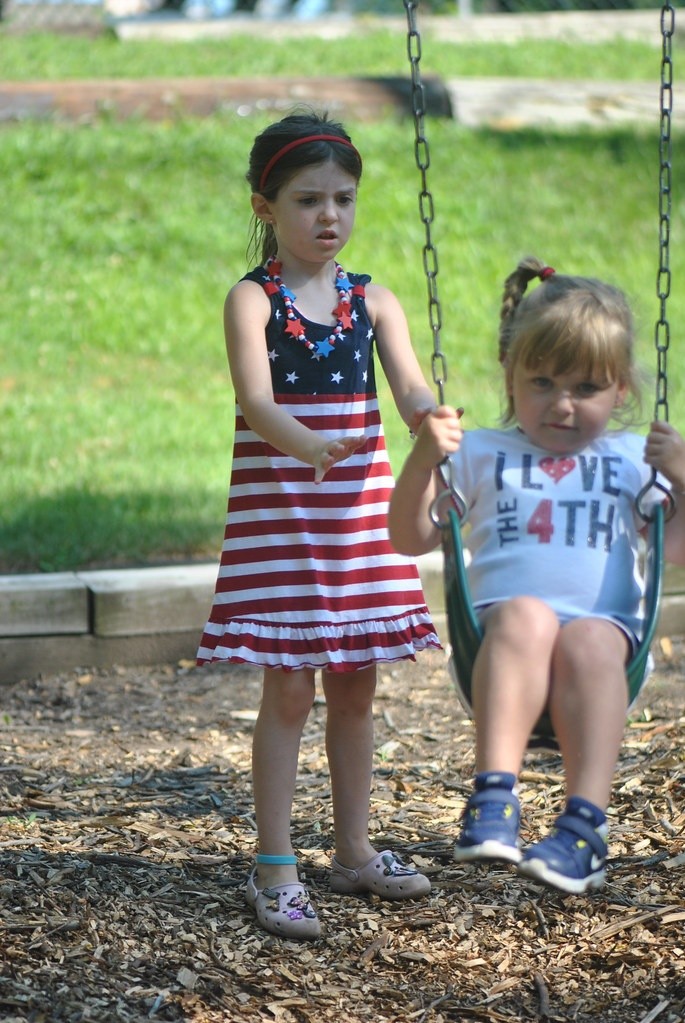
[268,255,354,358]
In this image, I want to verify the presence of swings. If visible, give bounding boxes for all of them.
[401,0,676,739]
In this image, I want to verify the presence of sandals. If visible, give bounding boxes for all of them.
[244,854,321,940]
[327,850,431,898]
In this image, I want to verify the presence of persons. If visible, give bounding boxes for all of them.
[194,114,441,941]
[386,255,685,892]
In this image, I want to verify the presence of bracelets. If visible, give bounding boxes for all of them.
[409,426,416,439]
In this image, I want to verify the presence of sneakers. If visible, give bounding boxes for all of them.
[453,771,523,864]
[518,795,609,893]
